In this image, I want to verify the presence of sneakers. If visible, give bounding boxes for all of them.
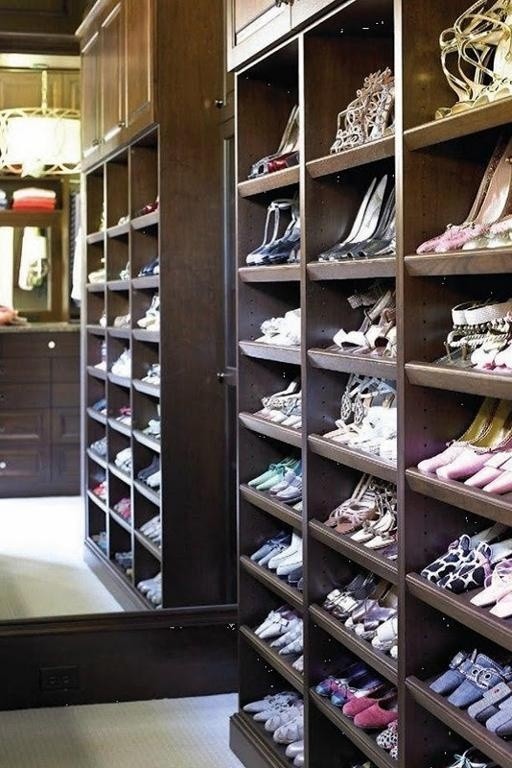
[312,647,510,768]
[88,195,159,611]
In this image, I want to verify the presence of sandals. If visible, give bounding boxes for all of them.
[432,289,512,374]
[329,67,395,154]
[435,0,512,121]
[331,282,397,359]
[322,376,397,647]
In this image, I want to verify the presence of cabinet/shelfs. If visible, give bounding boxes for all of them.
[1,333,81,499]
[74,0,225,172]
[71,128,226,611]
[217,0,509,768]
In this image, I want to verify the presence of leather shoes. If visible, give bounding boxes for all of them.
[243,383,306,767]
[408,398,512,624]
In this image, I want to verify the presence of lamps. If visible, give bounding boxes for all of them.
[0,69,82,179]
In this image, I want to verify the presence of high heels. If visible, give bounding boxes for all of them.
[245,191,301,265]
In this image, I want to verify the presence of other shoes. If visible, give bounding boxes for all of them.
[318,174,395,259]
[248,104,299,178]
[416,142,512,255]
[253,307,301,347]
